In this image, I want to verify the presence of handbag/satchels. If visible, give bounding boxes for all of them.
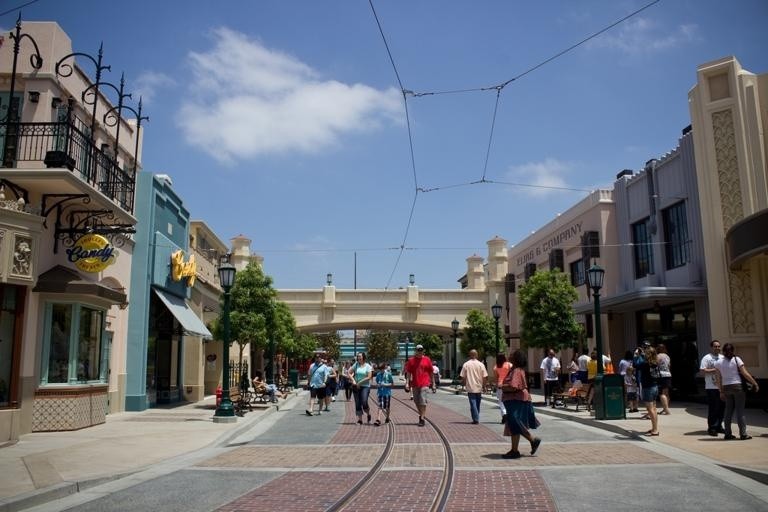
[740,374,755,394]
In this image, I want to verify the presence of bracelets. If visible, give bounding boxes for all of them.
[720,392,725,393]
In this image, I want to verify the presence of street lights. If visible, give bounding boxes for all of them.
[492,299,502,396]
[213,260,239,421]
[451,316,460,388]
[404,335,409,363]
[586,258,607,417]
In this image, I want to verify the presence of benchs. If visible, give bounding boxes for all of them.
[228,380,291,418]
[552,381,593,412]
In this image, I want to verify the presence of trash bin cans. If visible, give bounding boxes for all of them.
[527,373,541,388]
[594,374,626,418]
[288,370,299,387]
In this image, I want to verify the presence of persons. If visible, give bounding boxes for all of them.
[540,349,561,407]
[305,344,441,426]
[280,370,294,393]
[715,343,759,440]
[459,349,489,425]
[566,340,672,436]
[497,349,541,458]
[493,353,513,424]
[699,340,725,436]
[253,369,288,399]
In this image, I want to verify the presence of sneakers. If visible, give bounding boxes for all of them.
[500,450,521,460]
[708,425,725,436]
[530,436,541,456]
[501,414,507,425]
[284,392,288,399]
[628,407,673,420]
[305,407,330,416]
[724,435,736,440]
[418,416,426,427]
[331,396,352,402]
[356,415,391,426]
[740,435,752,440]
[470,420,480,424]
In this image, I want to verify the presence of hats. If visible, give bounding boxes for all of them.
[415,344,424,350]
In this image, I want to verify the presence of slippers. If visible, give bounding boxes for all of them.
[643,429,660,436]
